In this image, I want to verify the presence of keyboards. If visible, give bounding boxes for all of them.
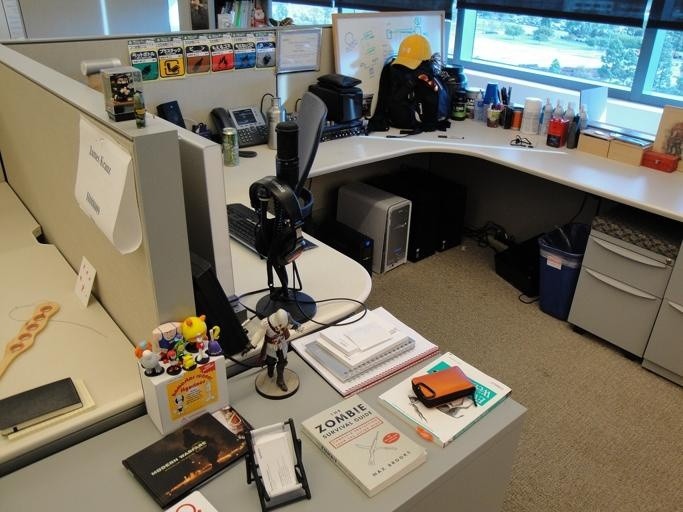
[227,202,268,260]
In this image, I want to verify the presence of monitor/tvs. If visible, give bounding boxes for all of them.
[146,110,236,297]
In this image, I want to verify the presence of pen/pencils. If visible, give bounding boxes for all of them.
[438,136,464,139]
[501,86,512,105]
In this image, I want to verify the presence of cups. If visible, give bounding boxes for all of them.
[482,82,524,130]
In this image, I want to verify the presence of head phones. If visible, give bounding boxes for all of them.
[248,175,306,267]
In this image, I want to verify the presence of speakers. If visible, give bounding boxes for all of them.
[327,219,374,278]
[157,100,186,129]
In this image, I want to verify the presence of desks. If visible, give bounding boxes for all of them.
[0,25,683,473]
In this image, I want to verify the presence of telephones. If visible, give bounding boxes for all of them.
[210,107,270,148]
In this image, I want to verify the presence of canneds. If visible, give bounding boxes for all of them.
[222,127,241,167]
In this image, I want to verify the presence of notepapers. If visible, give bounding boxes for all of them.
[344,316,393,352]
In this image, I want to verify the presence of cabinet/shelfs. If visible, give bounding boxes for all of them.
[0,349,528,512]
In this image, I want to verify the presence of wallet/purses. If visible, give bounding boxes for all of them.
[318,73,361,88]
[413,366,476,408]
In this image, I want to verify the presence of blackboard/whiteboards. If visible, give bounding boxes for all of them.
[332,10,445,99]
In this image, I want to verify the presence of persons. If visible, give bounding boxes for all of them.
[240,308,306,400]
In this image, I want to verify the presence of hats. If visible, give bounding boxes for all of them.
[390,35,432,70]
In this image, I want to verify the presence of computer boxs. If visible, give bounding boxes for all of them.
[337,181,413,273]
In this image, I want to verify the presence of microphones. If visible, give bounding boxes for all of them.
[275,121,301,188]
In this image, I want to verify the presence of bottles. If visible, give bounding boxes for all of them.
[473,92,484,121]
[450,89,467,121]
[539,97,588,149]
[267,98,286,150]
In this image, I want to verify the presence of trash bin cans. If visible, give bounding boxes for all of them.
[538,221,591,321]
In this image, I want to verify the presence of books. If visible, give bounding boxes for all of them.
[300,394,427,498]
[377,351,513,448]
[0,376,95,441]
[289,306,441,400]
[122,405,256,512]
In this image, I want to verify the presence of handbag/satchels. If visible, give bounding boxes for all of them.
[367,56,453,134]
